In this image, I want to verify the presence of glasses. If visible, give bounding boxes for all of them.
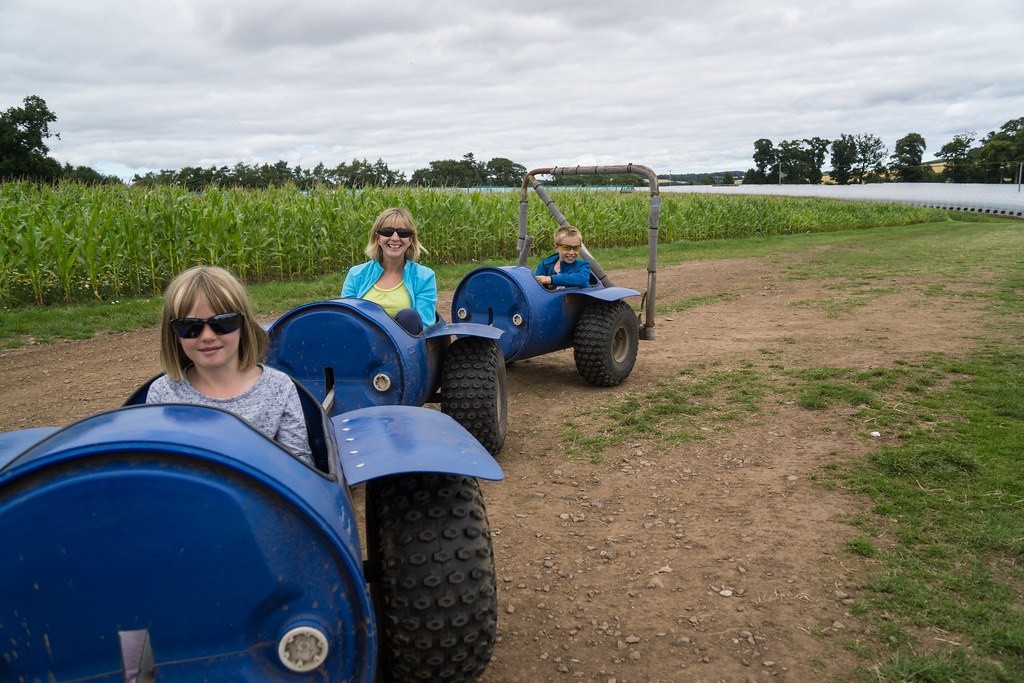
[376,227,414,238]
[168,312,244,338]
[556,243,582,252]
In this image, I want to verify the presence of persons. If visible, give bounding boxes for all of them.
[532,225,592,291]
[340,206,438,332]
[144,263,317,468]
